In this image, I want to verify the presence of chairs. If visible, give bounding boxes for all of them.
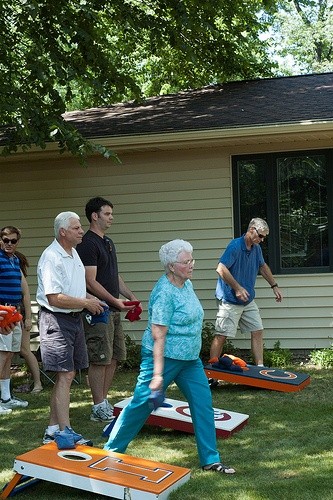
[28,301,79,389]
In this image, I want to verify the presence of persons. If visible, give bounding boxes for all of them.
[103,239,235,474]
[76,196,142,422]
[210,217,282,366]
[36,212,109,445]
[0,226,44,413]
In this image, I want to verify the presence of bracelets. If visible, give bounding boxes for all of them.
[271,283,278,288]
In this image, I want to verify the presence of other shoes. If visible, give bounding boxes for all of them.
[209,378,219,387]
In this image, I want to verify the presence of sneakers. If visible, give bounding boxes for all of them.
[0,398,28,408]
[54,426,93,447]
[0,404,12,414]
[90,400,116,422]
[43,429,81,445]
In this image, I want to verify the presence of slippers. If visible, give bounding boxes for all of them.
[31,387,44,393]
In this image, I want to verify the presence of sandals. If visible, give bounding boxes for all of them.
[202,462,235,474]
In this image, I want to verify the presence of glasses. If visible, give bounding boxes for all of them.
[253,226,266,238]
[2,237,18,244]
[176,258,196,265]
